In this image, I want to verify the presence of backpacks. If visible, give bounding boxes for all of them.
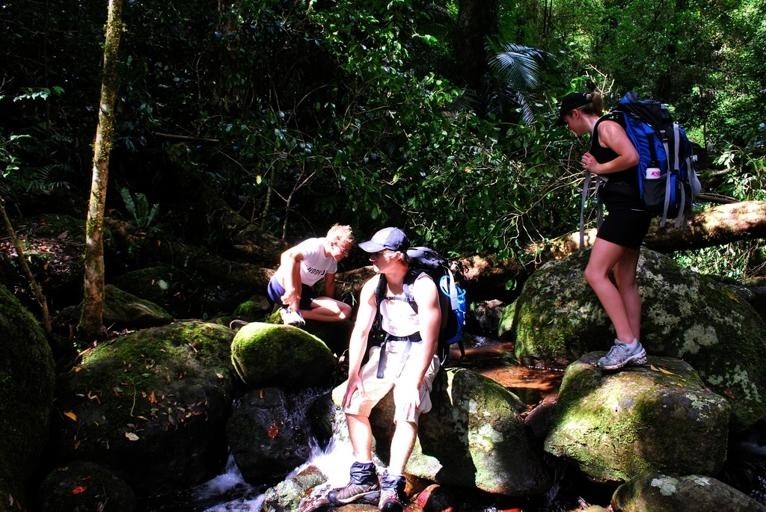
[593,92,702,219]
[371,246,466,360]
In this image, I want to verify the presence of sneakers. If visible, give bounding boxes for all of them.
[598,339,648,370]
[279,307,308,325]
[377,487,403,511]
[328,482,381,507]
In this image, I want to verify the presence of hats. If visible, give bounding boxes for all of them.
[555,92,592,127]
[358,227,410,254]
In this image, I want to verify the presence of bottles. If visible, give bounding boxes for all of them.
[645,159,661,183]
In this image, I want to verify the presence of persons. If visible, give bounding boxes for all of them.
[267,223,356,328]
[328,226,444,512]
[562,92,649,370]
